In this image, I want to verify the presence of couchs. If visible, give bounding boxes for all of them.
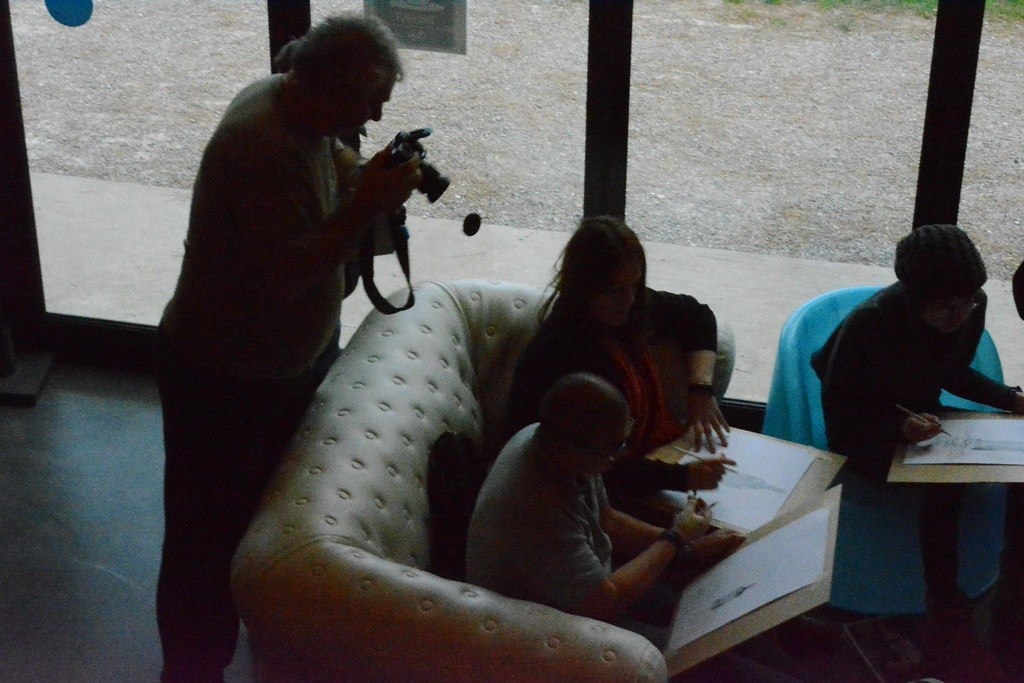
[229,279,736,683]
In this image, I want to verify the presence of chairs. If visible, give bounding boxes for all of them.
[764,285,1007,619]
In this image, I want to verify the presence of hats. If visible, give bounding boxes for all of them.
[894,223,987,298]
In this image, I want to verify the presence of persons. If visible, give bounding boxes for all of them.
[809,224,1024,620]
[463,371,746,629]
[143,11,426,683]
[503,216,738,506]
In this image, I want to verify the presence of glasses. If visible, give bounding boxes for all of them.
[925,300,980,315]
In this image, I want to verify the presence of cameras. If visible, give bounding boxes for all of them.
[382,126,481,237]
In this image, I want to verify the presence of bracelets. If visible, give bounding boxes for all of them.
[658,527,683,550]
[688,382,715,396]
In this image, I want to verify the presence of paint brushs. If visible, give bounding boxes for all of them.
[895,402,954,437]
[698,500,718,516]
[673,445,739,475]
